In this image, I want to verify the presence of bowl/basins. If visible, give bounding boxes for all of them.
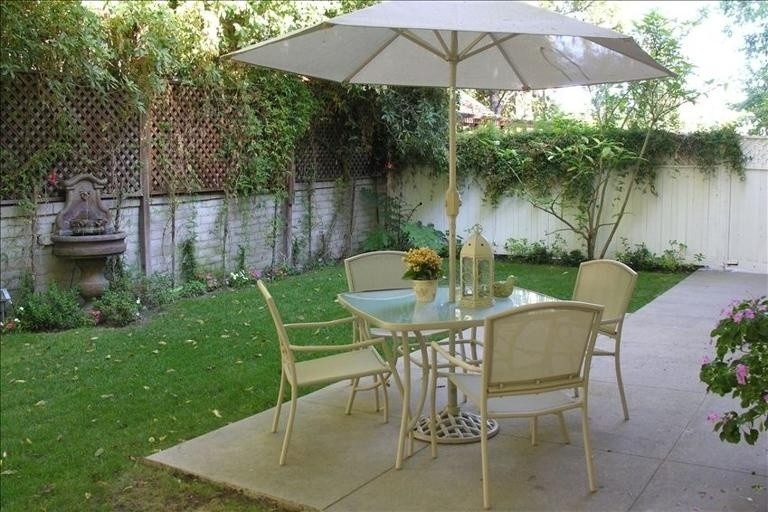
[492,281,513,298]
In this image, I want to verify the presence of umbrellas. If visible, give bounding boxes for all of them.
[216,1,682,413]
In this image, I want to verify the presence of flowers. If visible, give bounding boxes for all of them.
[697,291,768,446]
